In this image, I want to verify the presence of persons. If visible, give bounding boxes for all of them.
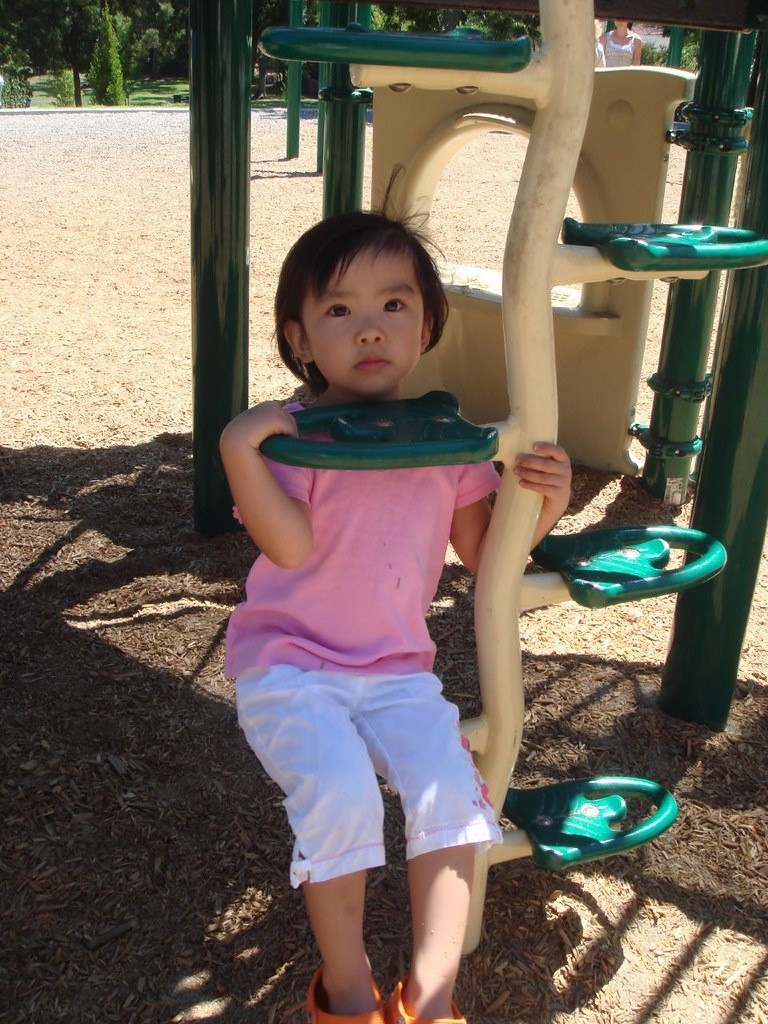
[218,210,573,1024]
[599,20,642,68]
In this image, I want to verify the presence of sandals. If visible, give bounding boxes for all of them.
[385,975,467,1024]
[304,963,385,1024]
